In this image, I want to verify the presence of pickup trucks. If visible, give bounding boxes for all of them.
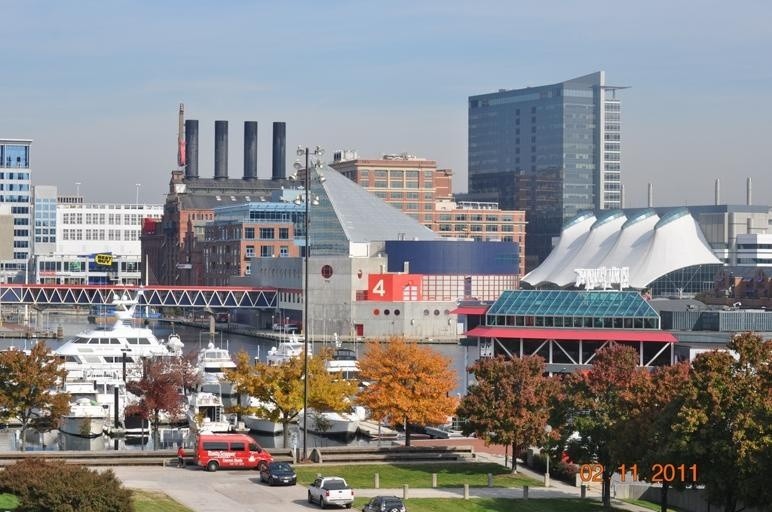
[308,476,355,510]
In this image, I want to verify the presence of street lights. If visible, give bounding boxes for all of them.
[288,144,327,464]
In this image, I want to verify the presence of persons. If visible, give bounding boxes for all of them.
[176,446,187,468]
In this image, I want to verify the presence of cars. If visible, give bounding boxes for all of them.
[259,461,298,487]
[362,495,407,512]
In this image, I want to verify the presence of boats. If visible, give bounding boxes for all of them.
[55,290,366,444]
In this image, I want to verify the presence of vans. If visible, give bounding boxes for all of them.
[195,433,273,472]
